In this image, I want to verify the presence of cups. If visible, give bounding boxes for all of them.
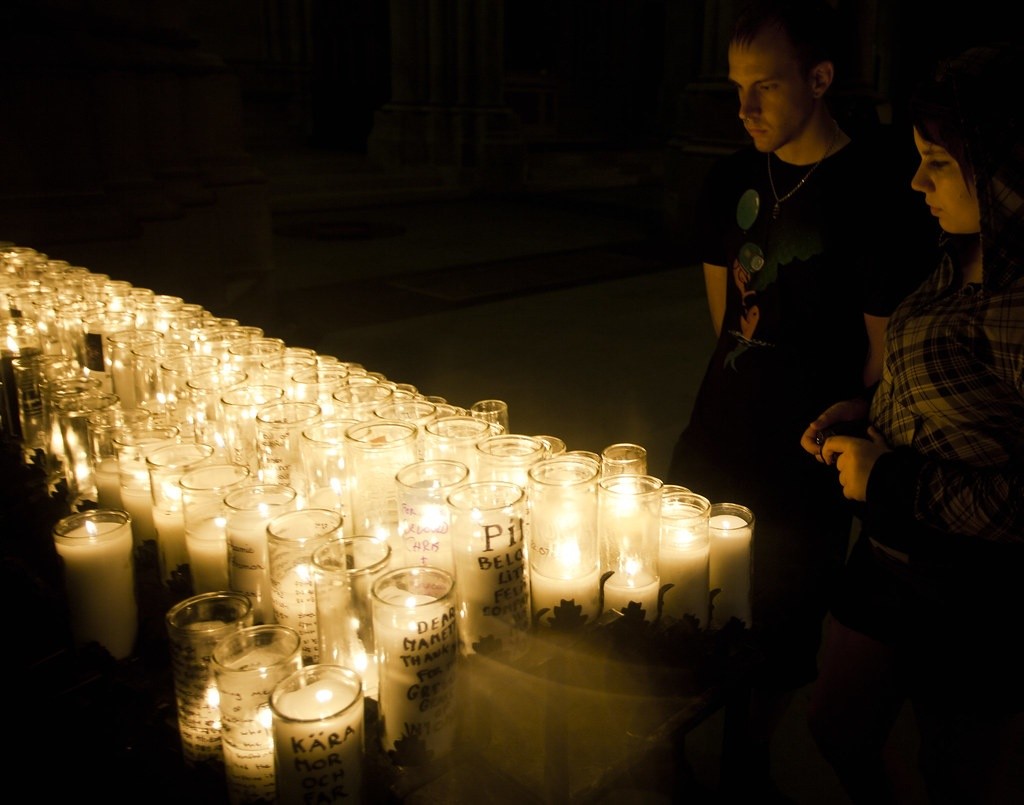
[1,242,754,804]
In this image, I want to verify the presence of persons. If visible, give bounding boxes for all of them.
[800,71,1022,804]
[664,1,916,805]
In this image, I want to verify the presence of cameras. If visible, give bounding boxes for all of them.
[817,420,874,464]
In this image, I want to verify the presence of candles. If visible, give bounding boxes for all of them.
[0,245,756,805]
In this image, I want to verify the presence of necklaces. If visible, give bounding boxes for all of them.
[766,117,839,221]
